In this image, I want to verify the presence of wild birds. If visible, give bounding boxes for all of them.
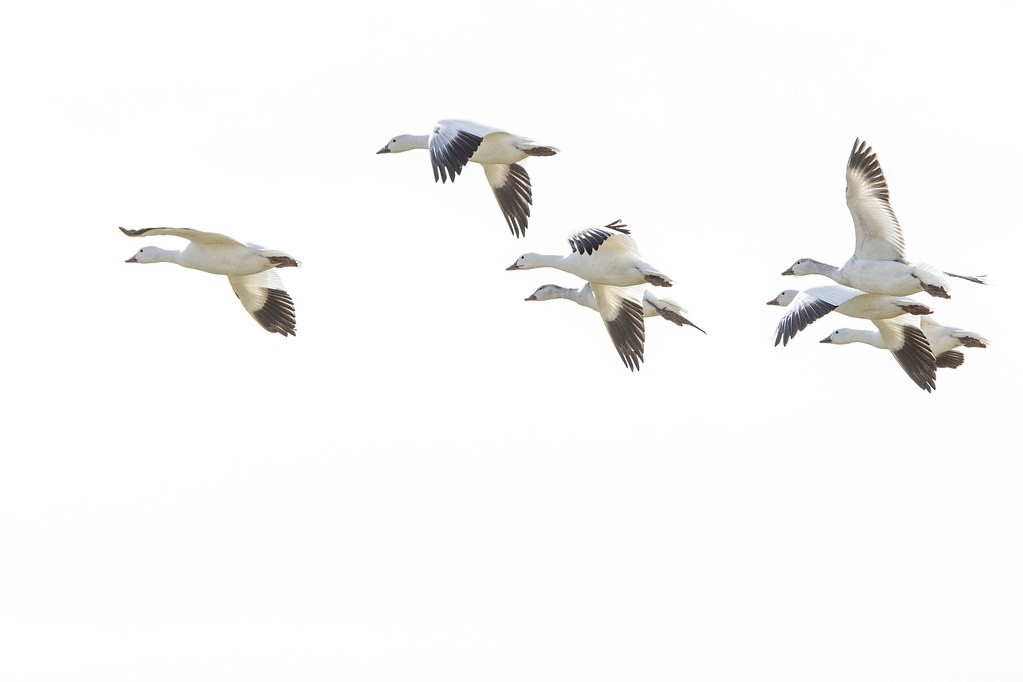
[118,226,300,338]
[505,219,708,372]
[766,137,990,394]
[376,119,559,240]
